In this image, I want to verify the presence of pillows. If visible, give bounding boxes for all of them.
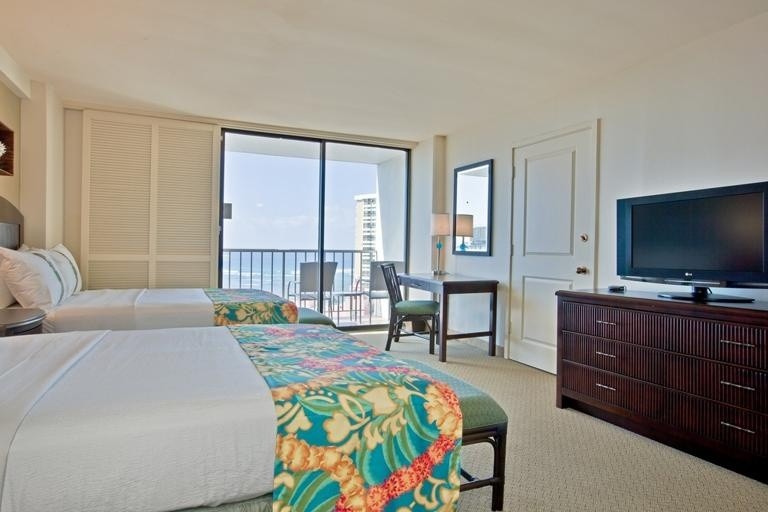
[0,242,29,312]
[47,243,82,297]
[0,244,69,316]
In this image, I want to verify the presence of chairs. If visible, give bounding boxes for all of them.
[287,261,339,320]
[380,262,440,354]
[355,261,402,325]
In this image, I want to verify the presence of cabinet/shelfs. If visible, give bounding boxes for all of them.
[555,289,768,485]
[0,120,14,176]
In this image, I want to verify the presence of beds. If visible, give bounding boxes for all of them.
[0,196,300,332]
[0,320,466,511]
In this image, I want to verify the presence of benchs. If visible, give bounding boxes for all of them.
[297,305,336,329]
[391,356,509,511]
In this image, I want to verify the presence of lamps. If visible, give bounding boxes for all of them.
[429,213,450,274]
[454,214,474,251]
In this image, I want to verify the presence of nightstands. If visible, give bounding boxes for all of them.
[0,305,48,336]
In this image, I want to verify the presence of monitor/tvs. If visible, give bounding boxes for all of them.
[615,181,768,302]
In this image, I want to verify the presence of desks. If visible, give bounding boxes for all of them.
[326,292,364,326]
[396,272,499,363]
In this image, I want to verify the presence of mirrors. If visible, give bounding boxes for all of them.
[452,158,493,257]
